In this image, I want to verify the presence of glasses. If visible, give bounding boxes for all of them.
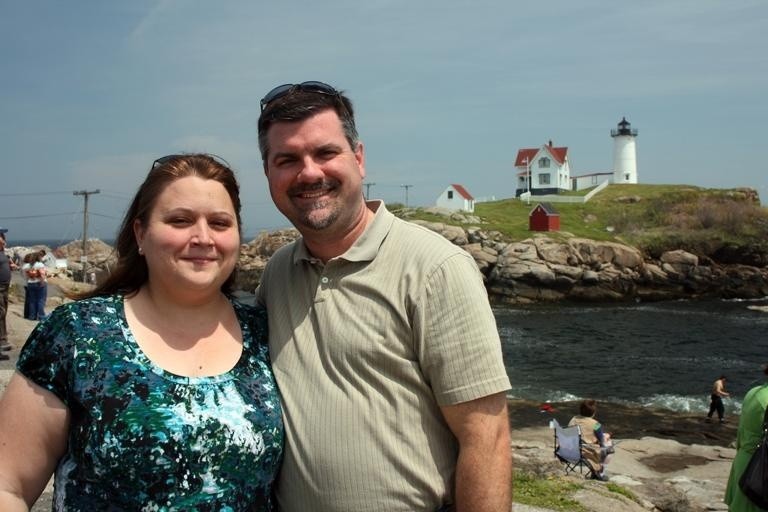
[258,80,345,115]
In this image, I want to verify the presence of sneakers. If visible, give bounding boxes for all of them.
[0,352,8,361]
[1,343,12,350]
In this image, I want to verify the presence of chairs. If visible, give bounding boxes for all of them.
[551,419,617,481]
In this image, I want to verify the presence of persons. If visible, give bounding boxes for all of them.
[567,400,615,481]
[705,375,729,423]
[722,382,768,511]
[0,154,286,512]
[0,229,52,361]
[248,82,514,511]
